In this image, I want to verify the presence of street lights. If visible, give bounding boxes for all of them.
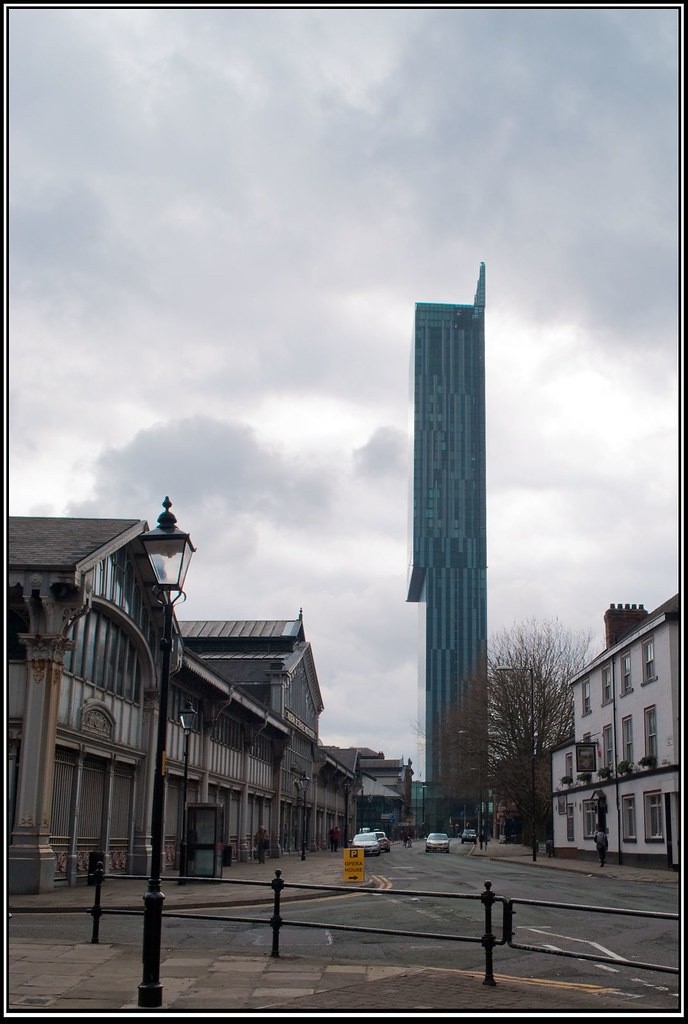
[127,495,200,1005]
[494,665,537,863]
[300,771,310,861]
[422,783,428,839]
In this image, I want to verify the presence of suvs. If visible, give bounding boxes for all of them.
[359,827,380,834]
[461,828,477,845]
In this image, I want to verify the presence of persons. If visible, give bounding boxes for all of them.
[329,826,334,852]
[255,826,268,865]
[333,826,340,852]
[594,828,608,867]
[400,830,413,848]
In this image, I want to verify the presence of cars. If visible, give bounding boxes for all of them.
[424,832,451,853]
[348,833,381,857]
[371,831,390,852]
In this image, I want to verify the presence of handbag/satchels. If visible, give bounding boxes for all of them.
[265,839,270,849]
[598,842,605,848]
